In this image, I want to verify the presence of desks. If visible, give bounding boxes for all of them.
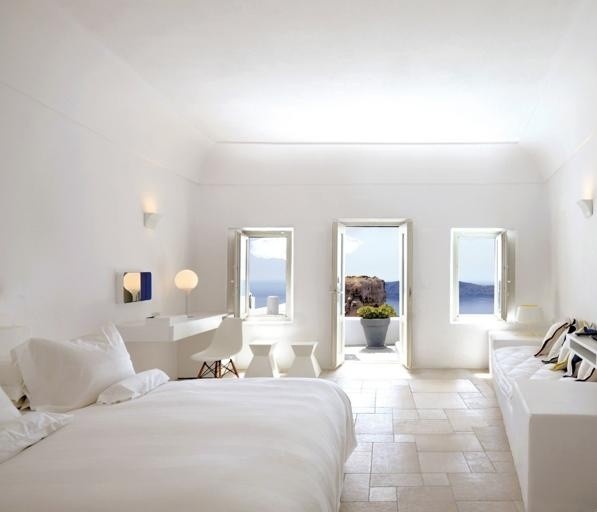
[114,311,228,381]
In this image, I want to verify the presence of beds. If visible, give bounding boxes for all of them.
[0,320,356,512]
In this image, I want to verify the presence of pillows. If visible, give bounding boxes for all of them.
[95,366,170,406]
[14,314,134,412]
[0,406,72,461]
[534,315,597,382]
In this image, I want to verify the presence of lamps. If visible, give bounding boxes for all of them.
[144,212,163,229]
[175,269,199,317]
[123,273,141,302]
[576,198,593,218]
[514,304,545,336]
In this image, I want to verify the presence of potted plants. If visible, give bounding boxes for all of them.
[358,303,397,348]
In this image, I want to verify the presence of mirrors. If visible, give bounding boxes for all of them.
[122,270,153,303]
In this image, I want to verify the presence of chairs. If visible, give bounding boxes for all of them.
[189,316,243,378]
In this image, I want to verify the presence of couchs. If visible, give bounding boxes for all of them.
[490,327,597,512]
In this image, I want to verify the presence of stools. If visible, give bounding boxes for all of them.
[286,341,322,378]
[244,336,280,378]
